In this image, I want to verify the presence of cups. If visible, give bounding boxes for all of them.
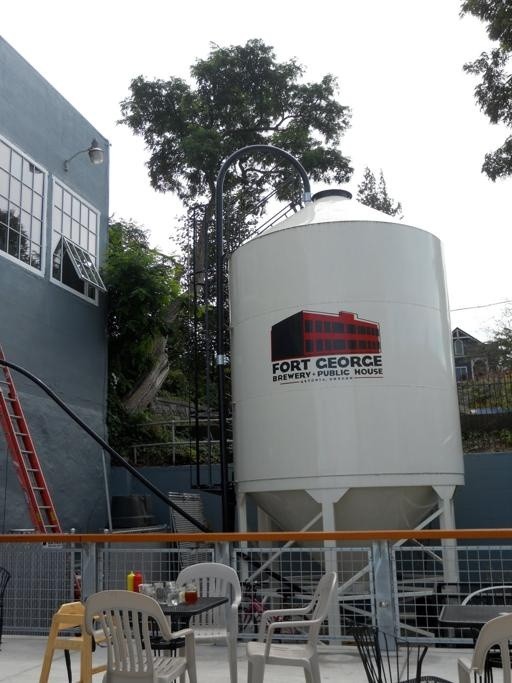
[138,581,199,607]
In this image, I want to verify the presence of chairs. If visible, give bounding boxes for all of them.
[453,611,511,682]
[348,623,437,683]
[458,583,512,606]
[83,588,200,683]
[241,570,340,681]
[42,600,124,682]
[168,562,242,682]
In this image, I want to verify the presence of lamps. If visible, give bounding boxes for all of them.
[64,138,104,171]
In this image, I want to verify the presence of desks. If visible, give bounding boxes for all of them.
[436,603,512,642]
[97,590,233,678]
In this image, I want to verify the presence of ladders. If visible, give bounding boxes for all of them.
[0,347,82,602]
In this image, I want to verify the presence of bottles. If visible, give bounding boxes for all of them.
[134,570,143,592]
[127,570,135,591]
